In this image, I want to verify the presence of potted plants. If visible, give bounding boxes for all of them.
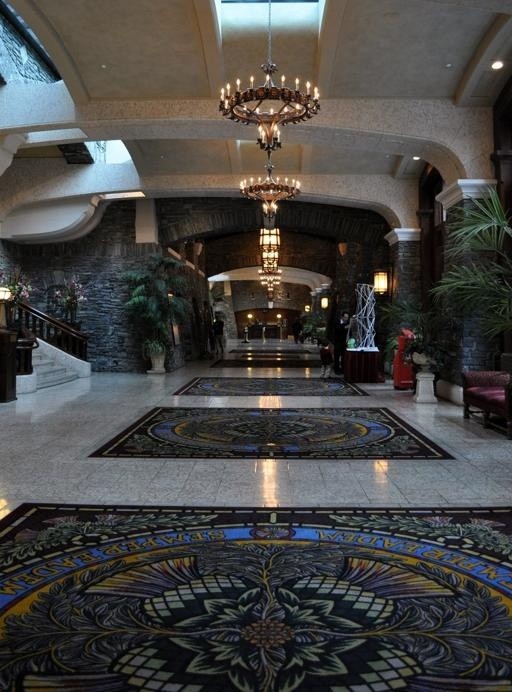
[116,253,192,374]
[40,280,85,334]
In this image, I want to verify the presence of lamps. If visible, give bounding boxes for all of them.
[257,228,282,310]
[304,305,310,313]
[219,3,320,160]
[239,160,302,226]
[373,271,388,295]
[320,298,328,308]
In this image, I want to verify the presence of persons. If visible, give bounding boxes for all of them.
[291,317,305,344]
[320,341,333,378]
[326,311,352,375]
[209,314,224,355]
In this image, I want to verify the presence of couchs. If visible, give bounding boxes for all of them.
[461,370,512,440]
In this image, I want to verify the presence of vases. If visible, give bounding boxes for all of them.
[16,339,36,375]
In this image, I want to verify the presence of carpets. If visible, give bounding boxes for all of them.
[0,501,512,692]
[208,349,323,368]
[172,376,370,397]
[87,405,455,461]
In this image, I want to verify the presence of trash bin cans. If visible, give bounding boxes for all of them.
[391,328,417,390]
[0,328,19,403]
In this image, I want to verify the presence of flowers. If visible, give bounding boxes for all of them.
[0,267,35,338]
